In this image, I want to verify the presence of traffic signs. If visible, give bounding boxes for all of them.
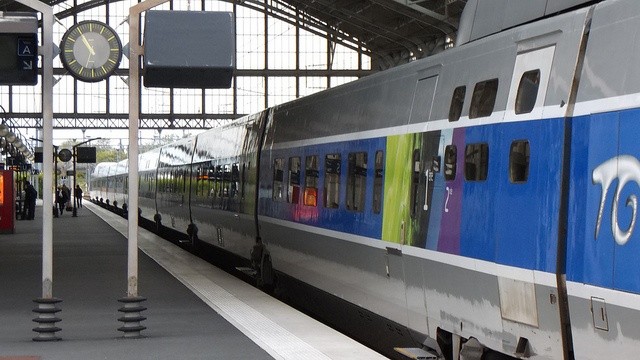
[0,32,37,86]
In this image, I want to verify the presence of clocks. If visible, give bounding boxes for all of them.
[60,148,72,162]
[60,20,122,83]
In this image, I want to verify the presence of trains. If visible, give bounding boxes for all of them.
[88,0,640,360]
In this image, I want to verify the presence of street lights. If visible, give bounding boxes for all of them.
[0,120,35,234]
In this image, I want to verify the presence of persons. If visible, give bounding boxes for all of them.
[22,177,37,220]
[55,182,70,215]
[75,185,82,208]
